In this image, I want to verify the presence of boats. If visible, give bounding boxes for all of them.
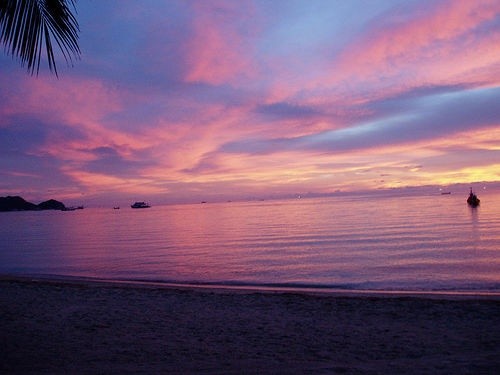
[72,205,84,209]
[467,187,480,209]
[129,201,152,208]
[60,206,77,211]
[114,206,119,209]
